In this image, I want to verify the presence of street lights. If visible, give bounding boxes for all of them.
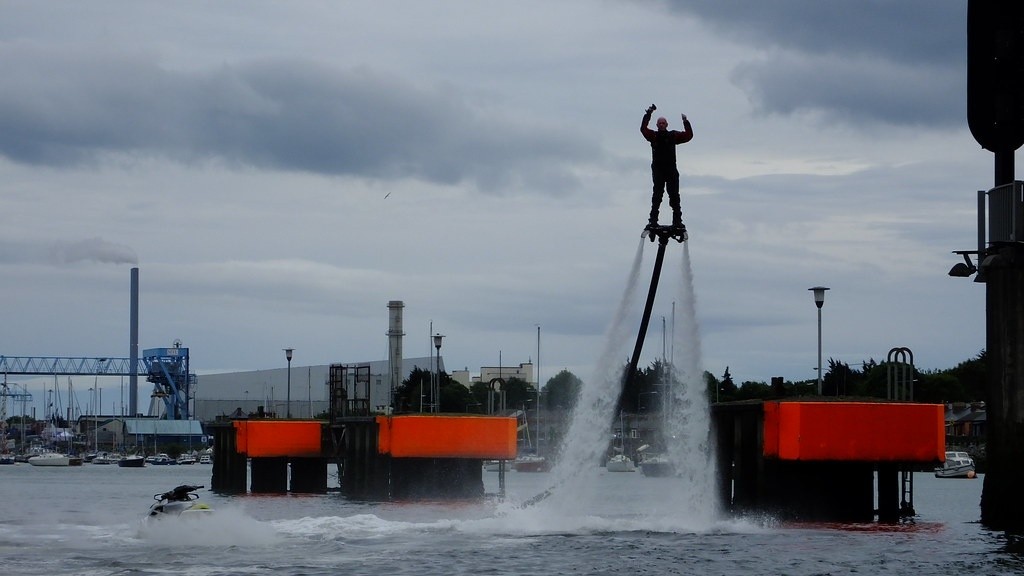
[282,347,295,419]
[807,286,831,397]
[430,332,446,414]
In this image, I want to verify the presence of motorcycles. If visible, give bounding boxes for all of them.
[147,482,216,520]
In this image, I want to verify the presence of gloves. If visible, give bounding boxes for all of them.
[645,105,654,115]
[681,113,688,121]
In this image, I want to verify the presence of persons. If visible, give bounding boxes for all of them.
[640,107,694,230]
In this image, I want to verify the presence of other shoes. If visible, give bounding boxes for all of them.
[673,219,686,228]
[650,219,658,228]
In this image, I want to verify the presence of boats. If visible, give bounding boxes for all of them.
[0,376,214,468]
[483,460,512,472]
[513,454,545,473]
[28,452,84,466]
[638,453,675,477]
[934,451,976,478]
[606,454,636,472]
[117,454,145,467]
